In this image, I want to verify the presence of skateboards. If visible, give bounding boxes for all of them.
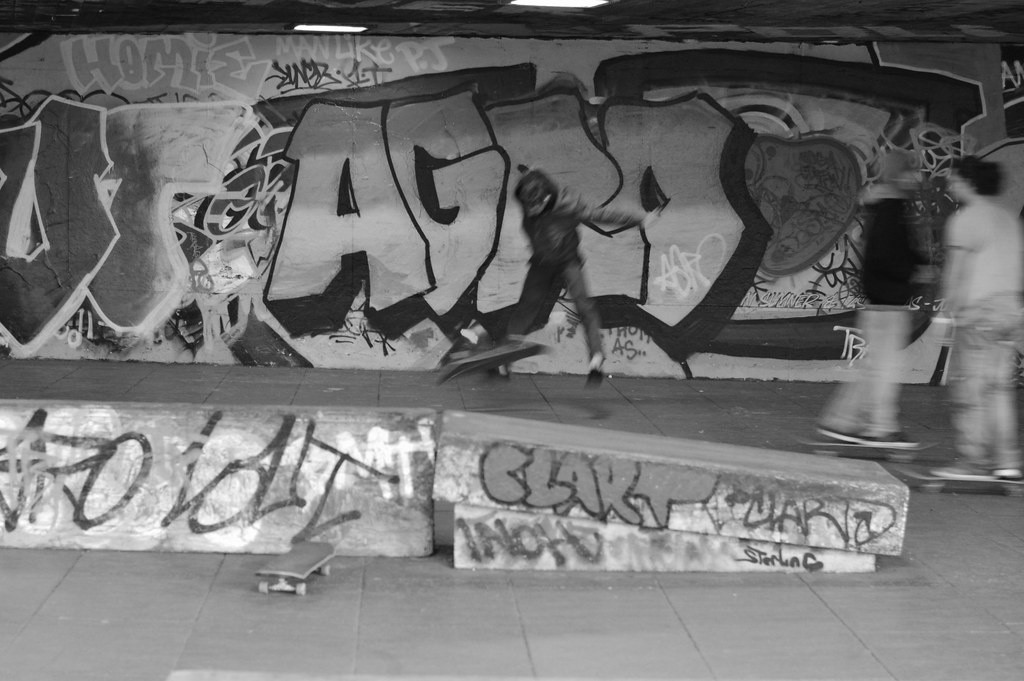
[884,461,1024,497]
[791,433,941,464]
[437,343,549,389]
[251,541,335,597]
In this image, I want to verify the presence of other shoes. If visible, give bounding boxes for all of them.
[859,430,919,449]
[991,466,1022,477]
[586,353,604,372]
[815,418,862,442]
[498,364,508,378]
[930,461,994,480]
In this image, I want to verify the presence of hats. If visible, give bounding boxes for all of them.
[878,150,920,181]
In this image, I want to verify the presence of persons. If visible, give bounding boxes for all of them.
[932,155,1023,485]
[819,145,934,448]
[462,172,656,388]
